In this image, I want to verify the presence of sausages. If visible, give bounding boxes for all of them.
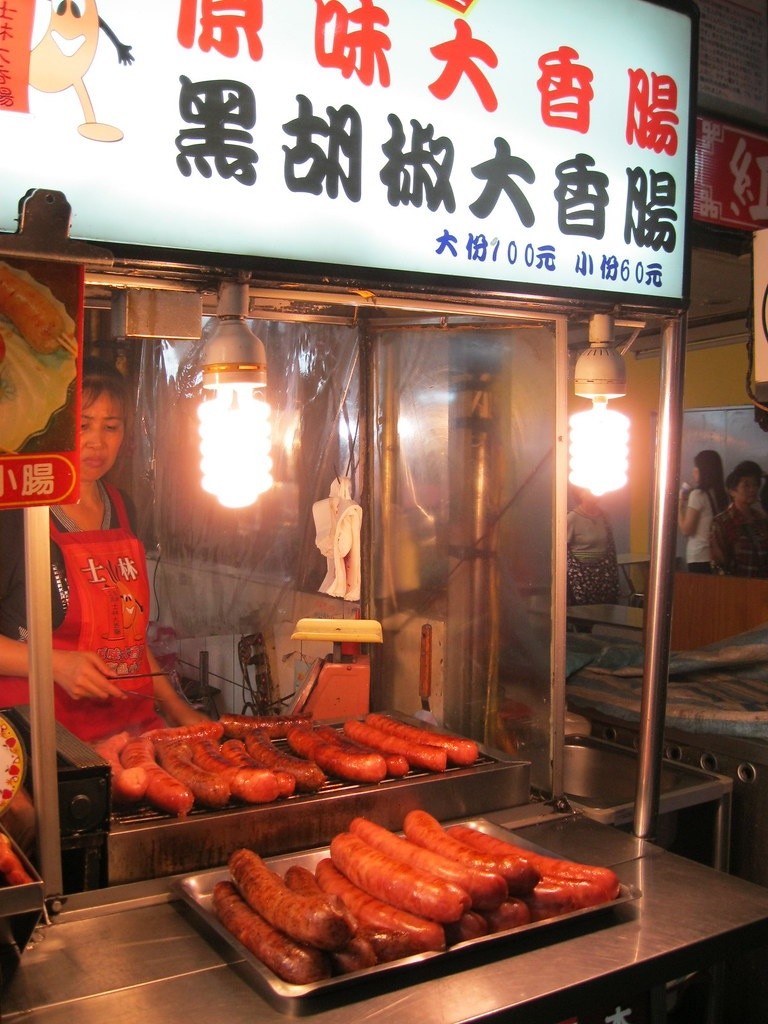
[92,712,480,817]
[0,832,36,885]
[213,811,623,985]
[0,260,65,355]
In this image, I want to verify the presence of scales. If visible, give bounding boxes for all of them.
[285,617,384,722]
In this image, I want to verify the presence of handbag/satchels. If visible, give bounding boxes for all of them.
[567,548,619,604]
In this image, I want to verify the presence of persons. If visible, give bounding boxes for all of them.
[566,486,619,633]
[735,460,768,519]
[0,355,212,746]
[676,450,732,575]
[709,467,768,580]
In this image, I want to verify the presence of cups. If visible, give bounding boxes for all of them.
[679,479,691,503]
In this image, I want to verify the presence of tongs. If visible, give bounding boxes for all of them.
[104,672,170,705]
